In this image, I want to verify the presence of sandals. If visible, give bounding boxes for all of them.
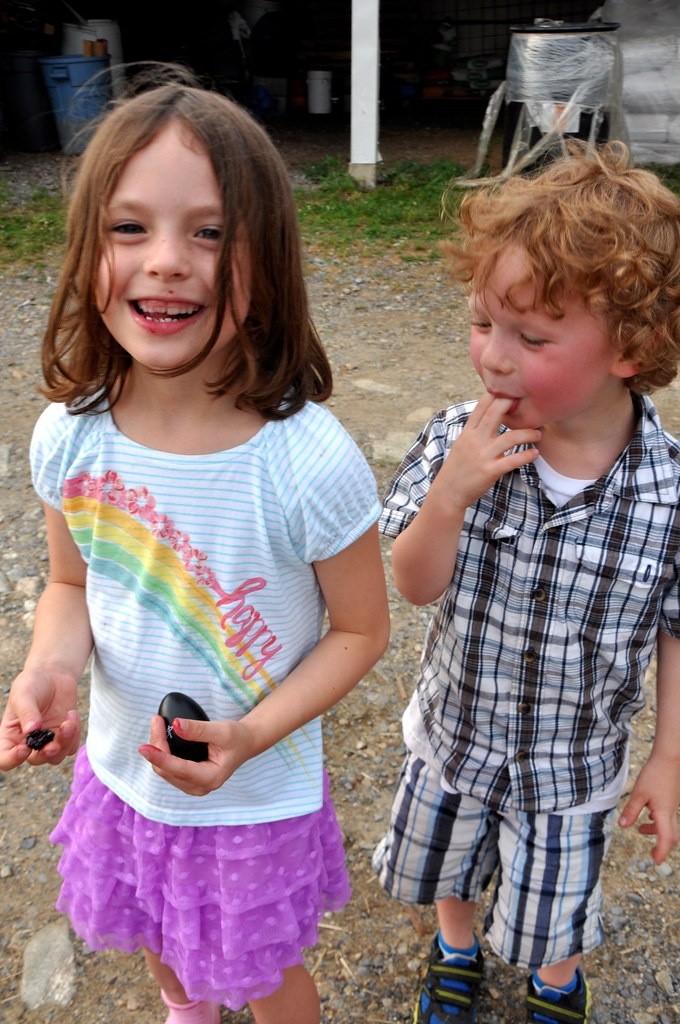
[524,966,591,1024]
[414,937,483,1024]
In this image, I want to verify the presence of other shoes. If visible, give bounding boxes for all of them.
[162,986,221,1024]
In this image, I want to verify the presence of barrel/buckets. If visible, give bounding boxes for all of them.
[308,71,331,113]
[0,48,63,153]
[39,54,114,156]
[503,23,620,174]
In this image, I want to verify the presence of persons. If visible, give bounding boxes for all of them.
[0,63,391,1024]
[371,137,680,1024]
